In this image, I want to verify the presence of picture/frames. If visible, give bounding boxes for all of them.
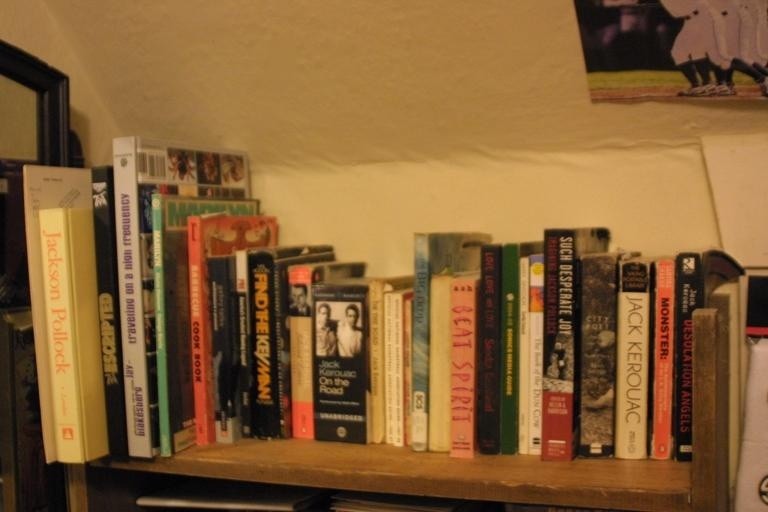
[572,1,768,105]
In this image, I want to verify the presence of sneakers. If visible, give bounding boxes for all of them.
[760,77,768,96]
[676,83,738,97]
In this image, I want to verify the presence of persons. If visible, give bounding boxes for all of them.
[658,0,768,98]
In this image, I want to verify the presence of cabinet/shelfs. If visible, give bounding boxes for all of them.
[63,275,749,512]
